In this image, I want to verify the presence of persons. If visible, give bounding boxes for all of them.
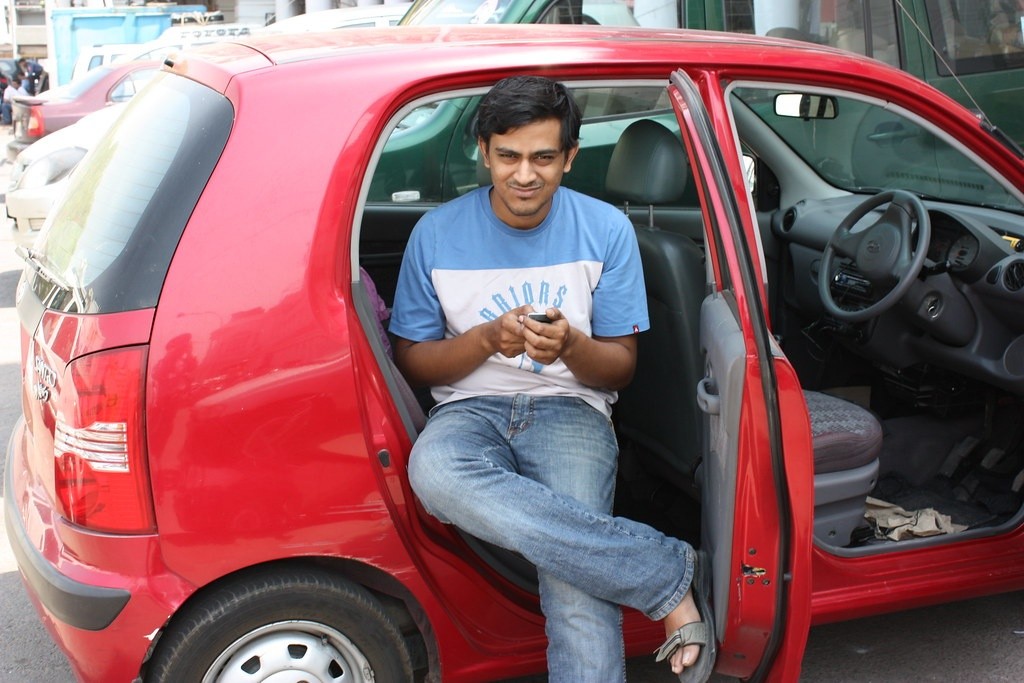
[0,57,44,127]
[386,77,717,683]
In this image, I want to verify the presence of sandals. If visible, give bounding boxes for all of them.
[654,550,717,683]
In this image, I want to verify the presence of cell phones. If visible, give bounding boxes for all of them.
[527,313,553,324]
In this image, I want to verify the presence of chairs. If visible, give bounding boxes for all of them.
[605,119,882,543]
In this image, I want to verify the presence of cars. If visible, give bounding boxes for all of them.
[0,21,1024,683]
[4,0,1024,271]
